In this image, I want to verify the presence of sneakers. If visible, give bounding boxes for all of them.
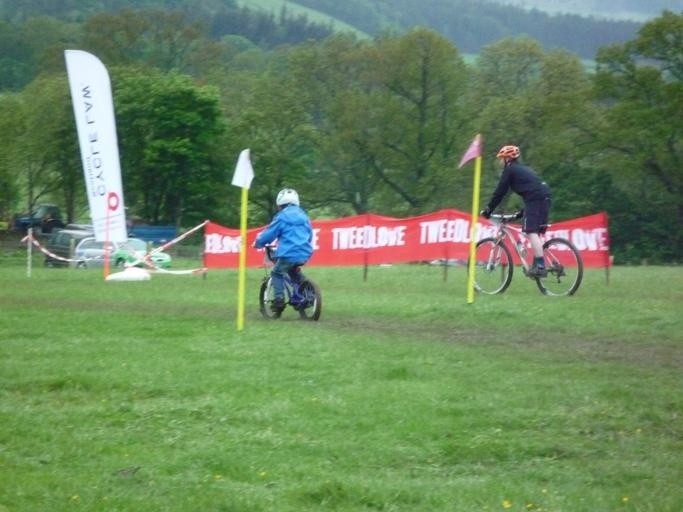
[527,263,545,276]
[270,299,284,307]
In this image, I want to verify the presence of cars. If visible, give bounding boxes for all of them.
[12,203,66,233]
[43,224,177,269]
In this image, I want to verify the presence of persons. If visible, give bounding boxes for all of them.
[482,145,553,278]
[250,187,314,310]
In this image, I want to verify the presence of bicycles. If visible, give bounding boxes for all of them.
[467,211,583,296]
[254,243,322,321]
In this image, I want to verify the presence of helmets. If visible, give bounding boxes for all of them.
[496,145,520,159]
[276,188,299,206]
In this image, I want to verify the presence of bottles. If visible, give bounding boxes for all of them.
[517,241,526,257]
